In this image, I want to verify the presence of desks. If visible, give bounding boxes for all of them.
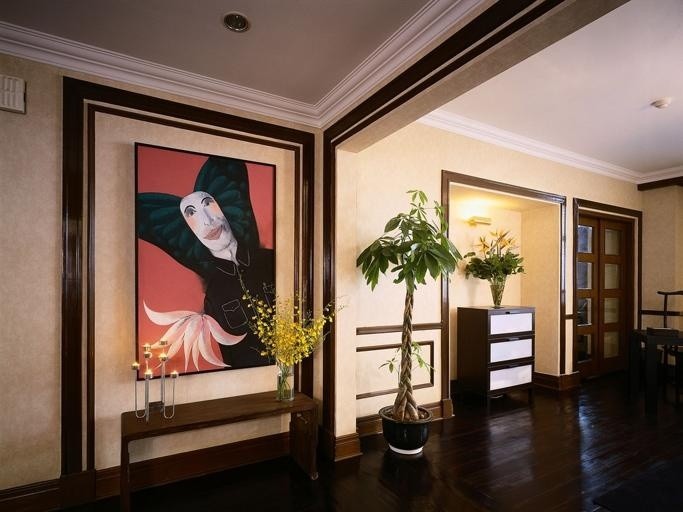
[120,386,320,511]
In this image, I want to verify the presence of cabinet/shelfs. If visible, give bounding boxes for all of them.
[456,305,536,419]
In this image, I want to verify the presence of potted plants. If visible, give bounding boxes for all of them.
[356,188,463,458]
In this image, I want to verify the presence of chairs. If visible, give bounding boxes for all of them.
[626,289,682,418]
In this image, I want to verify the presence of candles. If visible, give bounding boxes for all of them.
[131,338,180,380]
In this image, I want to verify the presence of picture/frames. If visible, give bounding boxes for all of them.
[58,72,317,477]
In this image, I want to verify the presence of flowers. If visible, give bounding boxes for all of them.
[236,281,350,398]
[462,226,529,308]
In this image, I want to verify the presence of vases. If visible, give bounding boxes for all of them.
[276,371,293,402]
[485,278,506,307]
